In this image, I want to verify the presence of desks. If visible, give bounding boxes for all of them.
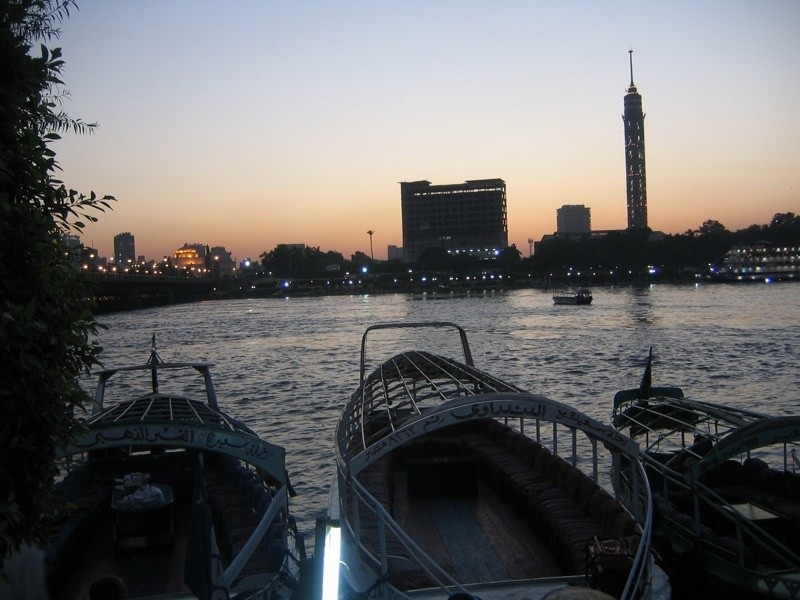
[111,485,175,561]
[720,501,787,569]
[404,453,479,501]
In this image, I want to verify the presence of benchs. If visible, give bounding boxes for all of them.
[22,446,284,600]
[355,407,644,592]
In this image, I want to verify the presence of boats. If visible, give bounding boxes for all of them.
[611,346,798,599]
[333,323,800,600]
[552,289,593,305]
[0,334,305,600]
[696,245,800,283]
